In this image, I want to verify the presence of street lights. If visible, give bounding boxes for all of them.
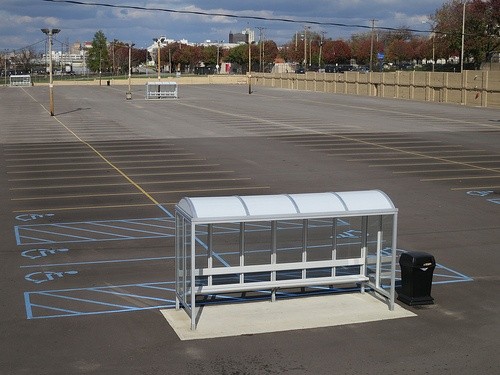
[152,37,166,98]
[422,20,437,73]
[111,39,118,76]
[124,43,135,99]
[40,28,61,115]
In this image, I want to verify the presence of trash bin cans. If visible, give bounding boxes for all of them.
[107,81,110,87]
[400,250,434,306]
